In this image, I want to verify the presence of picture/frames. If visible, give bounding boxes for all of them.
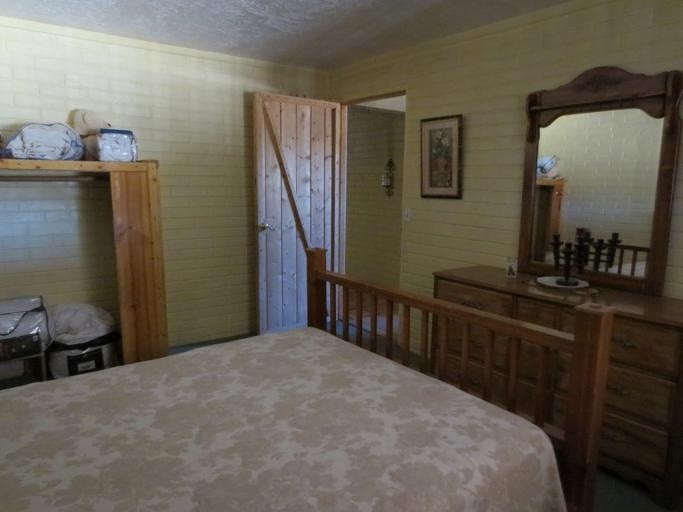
[417,113,464,198]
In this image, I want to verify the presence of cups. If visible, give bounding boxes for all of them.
[505,255,518,278]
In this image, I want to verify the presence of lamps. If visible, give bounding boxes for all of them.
[380,159,397,198]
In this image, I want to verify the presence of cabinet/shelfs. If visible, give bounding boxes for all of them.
[431,264,683,511]
[0,157,172,369]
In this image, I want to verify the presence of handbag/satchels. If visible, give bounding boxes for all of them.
[74,110,111,136]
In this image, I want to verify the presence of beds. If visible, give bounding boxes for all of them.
[0,246,615,512]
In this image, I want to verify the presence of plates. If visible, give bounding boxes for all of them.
[537,275,589,290]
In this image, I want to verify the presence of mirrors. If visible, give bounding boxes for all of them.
[515,63,682,298]
